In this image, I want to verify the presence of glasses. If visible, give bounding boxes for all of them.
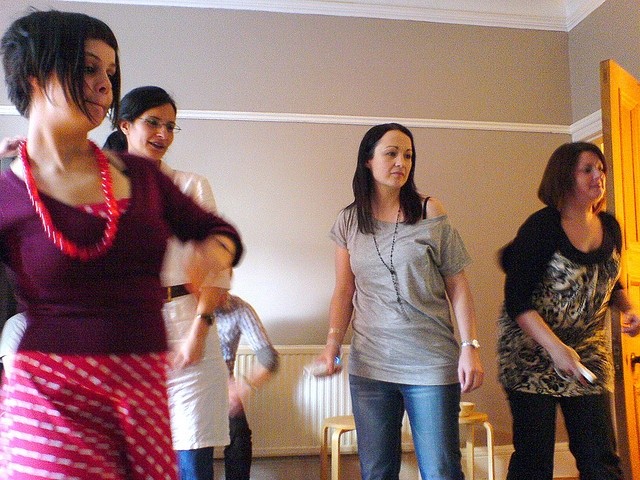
[137,115,181,133]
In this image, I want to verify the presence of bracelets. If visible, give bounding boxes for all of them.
[326,327,346,336]
[458,339,481,350]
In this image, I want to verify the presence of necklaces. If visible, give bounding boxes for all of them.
[17,140,121,264]
[366,195,413,323]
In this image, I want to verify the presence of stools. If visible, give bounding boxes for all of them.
[458,412,495,479]
[320,417,359,479]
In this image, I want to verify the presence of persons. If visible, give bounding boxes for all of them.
[496,142,640,480]
[0,8,243,480]
[203,266,281,479]
[95,87,232,480]
[316,123,484,479]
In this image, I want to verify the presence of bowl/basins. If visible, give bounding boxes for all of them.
[459,402,476,417]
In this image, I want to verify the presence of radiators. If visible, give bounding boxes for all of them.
[212,344,416,459]
